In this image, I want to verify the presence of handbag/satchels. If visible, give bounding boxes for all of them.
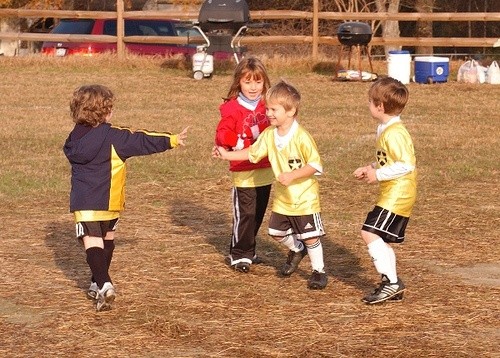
[456,59,485,84]
[484,59,500,84]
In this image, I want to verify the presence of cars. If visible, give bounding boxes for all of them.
[41,17,243,62]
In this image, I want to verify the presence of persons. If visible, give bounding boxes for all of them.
[216,57,274,273]
[212,78,328,289]
[351,77,417,305]
[62,85,190,312]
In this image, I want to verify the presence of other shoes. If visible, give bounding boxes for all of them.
[87,282,101,300]
[253,254,273,264]
[95,281,116,312]
[234,262,250,273]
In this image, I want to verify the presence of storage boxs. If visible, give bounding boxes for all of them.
[414,55,450,83]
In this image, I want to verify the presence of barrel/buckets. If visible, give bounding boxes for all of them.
[387,50,411,85]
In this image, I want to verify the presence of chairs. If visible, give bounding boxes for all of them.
[192,0,250,64]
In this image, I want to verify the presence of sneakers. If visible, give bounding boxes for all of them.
[361,274,405,305]
[281,239,308,275]
[308,270,327,290]
